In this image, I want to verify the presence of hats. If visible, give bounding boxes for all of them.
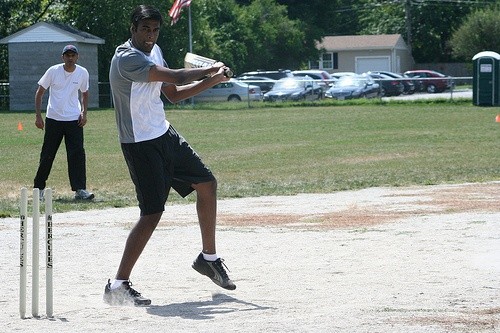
[63,45,78,55]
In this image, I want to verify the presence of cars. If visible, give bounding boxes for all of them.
[185,77,261,104]
[232,68,454,104]
[262,75,323,103]
[323,75,380,99]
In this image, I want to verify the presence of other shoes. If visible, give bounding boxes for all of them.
[74,190,94,200]
[38,190,45,201]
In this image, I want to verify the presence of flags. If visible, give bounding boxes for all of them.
[169,0,192,24]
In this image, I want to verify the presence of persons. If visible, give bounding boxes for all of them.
[33,45,95,200]
[103,5,236,306]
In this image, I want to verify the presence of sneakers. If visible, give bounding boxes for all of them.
[104,278,151,306]
[191,252,237,292]
